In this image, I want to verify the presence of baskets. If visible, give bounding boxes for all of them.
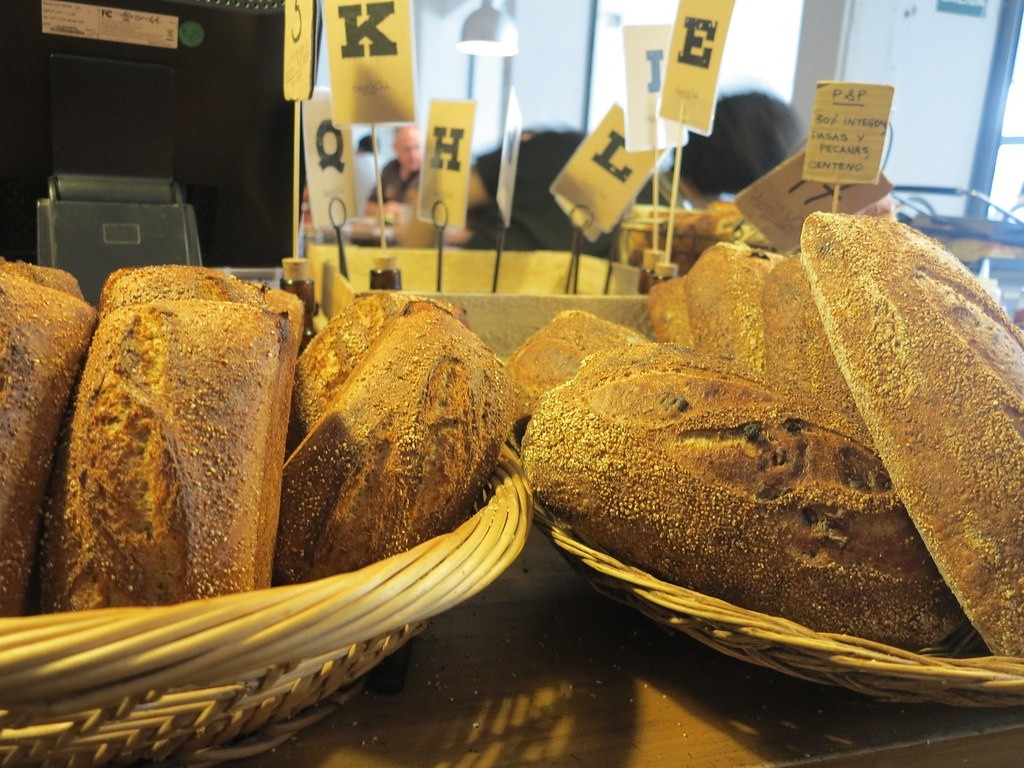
[507,430,1024,708]
[2,442,536,768]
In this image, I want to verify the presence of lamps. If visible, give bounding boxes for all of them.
[455,1,518,57]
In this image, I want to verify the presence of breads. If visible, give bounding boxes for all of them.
[0,262,515,618]
[501,200,1024,665]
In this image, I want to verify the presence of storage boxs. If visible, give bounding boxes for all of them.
[309,244,651,363]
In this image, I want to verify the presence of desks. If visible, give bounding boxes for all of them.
[230,521,1024,768]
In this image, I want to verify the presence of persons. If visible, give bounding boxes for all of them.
[468,128,594,250]
[366,126,422,227]
[355,135,388,215]
[581,91,811,258]
